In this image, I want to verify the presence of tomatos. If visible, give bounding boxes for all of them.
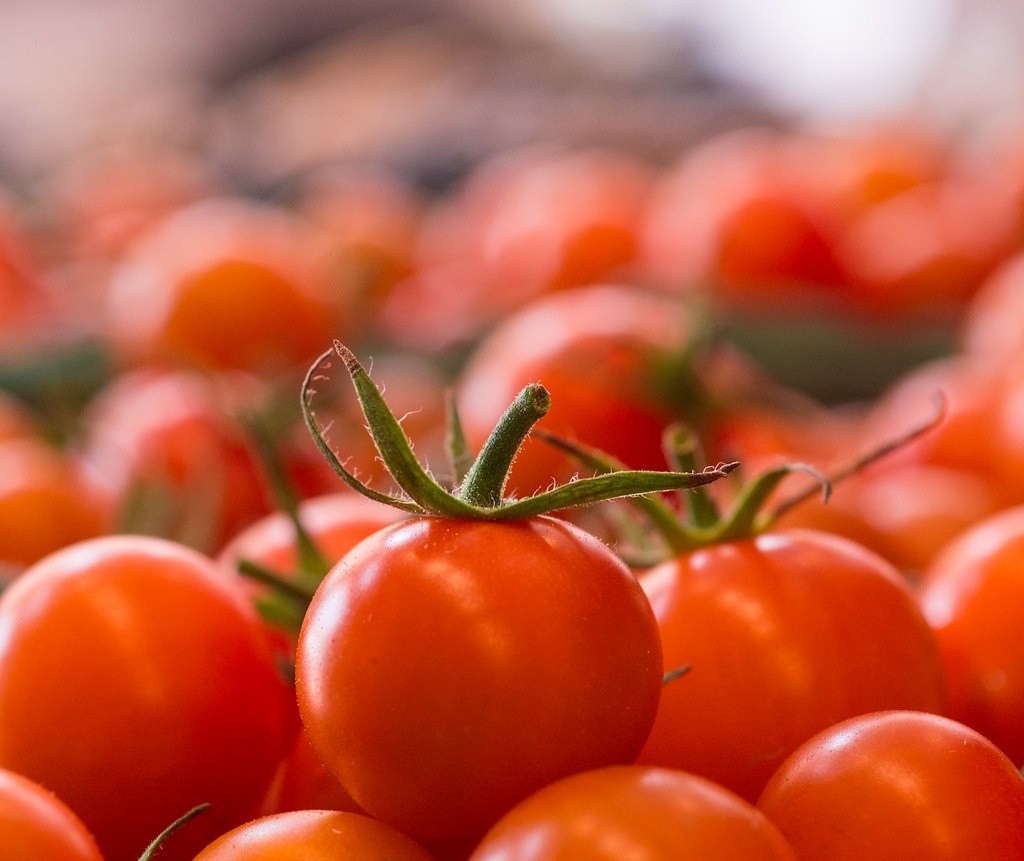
[0,115,1024,861]
